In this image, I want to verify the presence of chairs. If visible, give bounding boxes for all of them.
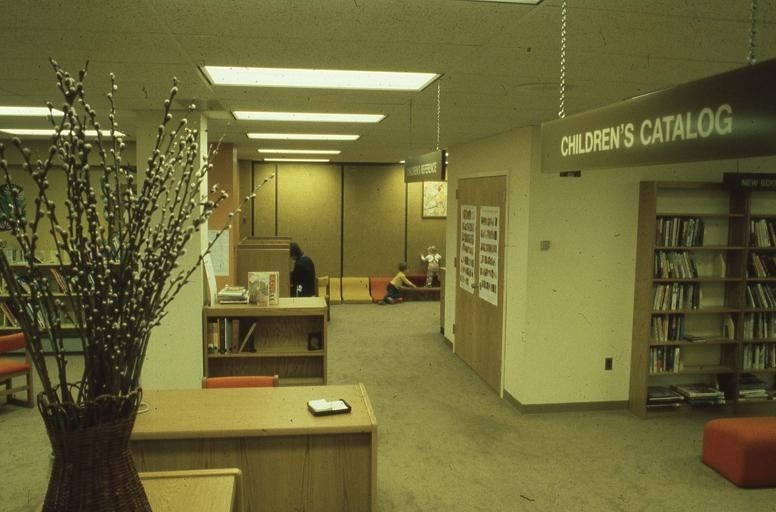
[315,278,330,321]
[202,375,278,386]
[0,333,34,408]
[369,276,404,304]
[341,276,373,304]
[329,277,342,305]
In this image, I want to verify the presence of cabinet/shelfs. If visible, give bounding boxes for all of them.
[0,263,97,330]
[627,180,776,420]
[203,298,328,385]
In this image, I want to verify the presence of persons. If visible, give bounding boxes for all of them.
[418,245,443,289]
[377,261,416,305]
[287,242,316,296]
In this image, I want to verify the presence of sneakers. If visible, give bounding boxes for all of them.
[378,297,394,304]
[424,285,432,288]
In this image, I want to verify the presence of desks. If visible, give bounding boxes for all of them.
[126,382,378,512]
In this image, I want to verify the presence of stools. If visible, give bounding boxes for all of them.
[702,416,776,489]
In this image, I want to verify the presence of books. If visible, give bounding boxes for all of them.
[205,271,280,355]
[0,241,94,329]
[646,214,776,408]
